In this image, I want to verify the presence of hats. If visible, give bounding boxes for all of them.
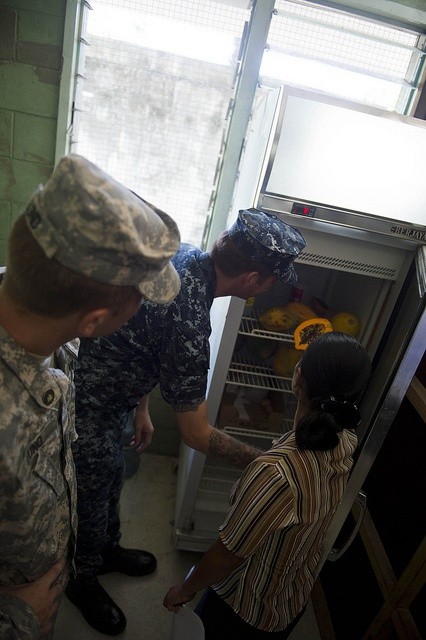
[21,153,182,305]
[227,209,307,287]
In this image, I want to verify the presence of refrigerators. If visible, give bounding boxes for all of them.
[175,190,425,563]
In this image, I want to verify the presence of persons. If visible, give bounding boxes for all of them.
[163,333,374,640]
[0,155,182,639]
[65,209,306,635]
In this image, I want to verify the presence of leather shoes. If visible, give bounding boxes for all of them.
[73,546,156,577]
[64,574,128,636]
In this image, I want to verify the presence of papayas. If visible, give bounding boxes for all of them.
[332,310,361,337]
[283,302,317,322]
[259,309,298,331]
[294,317,333,351]
[272,349,302,376]
